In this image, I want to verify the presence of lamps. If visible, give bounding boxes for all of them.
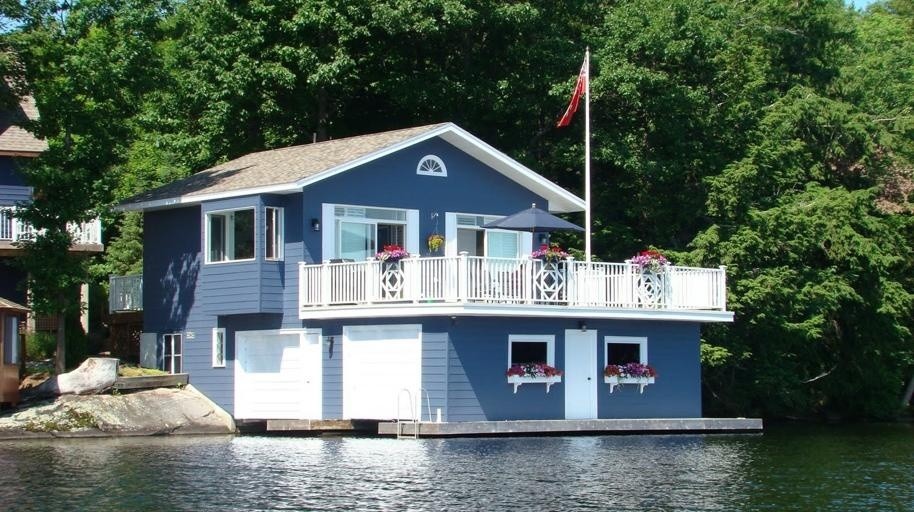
[327,336,334,346]
[312,218,321,232]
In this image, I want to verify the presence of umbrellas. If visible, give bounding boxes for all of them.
[481,203,585,258]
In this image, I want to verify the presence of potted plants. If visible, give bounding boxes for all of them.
[428,234,445,253]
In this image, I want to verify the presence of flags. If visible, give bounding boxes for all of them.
[559,54,586,127]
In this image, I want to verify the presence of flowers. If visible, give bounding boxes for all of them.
[604,363,658,383]
[529,243,571,263]
[630,248,670,274]
[375,243,409,262]
[505,360,563,380]
